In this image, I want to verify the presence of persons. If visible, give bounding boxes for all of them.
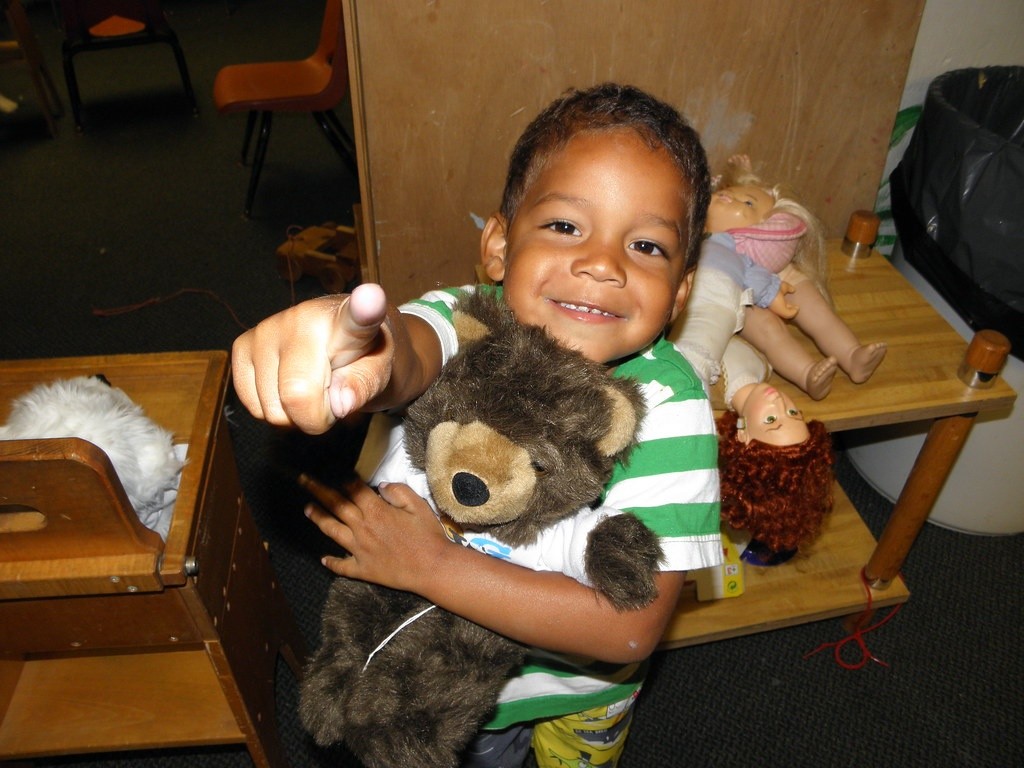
[674,154,823,386]
[733,266,888,402]
[230,79,728,768]
[667,309,839,549]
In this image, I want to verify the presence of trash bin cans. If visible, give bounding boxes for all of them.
[844,65,1024,539]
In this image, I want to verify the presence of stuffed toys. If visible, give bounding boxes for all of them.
[299,279,665,768]
[1,373,191,532]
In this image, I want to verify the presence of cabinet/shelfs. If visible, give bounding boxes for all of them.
[472,209,1017,660]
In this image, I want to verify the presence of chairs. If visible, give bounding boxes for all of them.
[0,0,65,142]
[56,0,199,137]
[213,0,356,224]
[0,350,307,768]
